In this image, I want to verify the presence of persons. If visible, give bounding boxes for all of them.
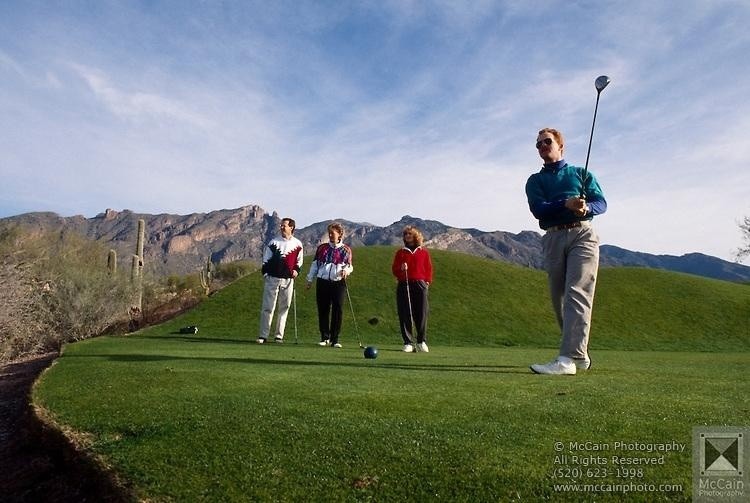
[255,218,303,344]
[306,223,354,348]
[525,128,607,374]
[393,226,432,352]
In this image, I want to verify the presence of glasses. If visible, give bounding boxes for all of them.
[536,138,552,149]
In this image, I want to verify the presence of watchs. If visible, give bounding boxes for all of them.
[586,204,590,214]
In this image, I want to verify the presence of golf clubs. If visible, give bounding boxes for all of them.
[293,289,298,342]
[404,263,419,353]
[580,76,610,198]
[344,277,366,348]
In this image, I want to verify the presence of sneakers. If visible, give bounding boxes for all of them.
[574,349,591,369]
[329,342,342,348]
[530,360,576,375]
[416,341,429,352]
[256,339,266,344]
[318,339,329,346]
[402,344,414,352]
[274,338,283,344]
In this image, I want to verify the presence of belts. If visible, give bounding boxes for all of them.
[557,222,580,230]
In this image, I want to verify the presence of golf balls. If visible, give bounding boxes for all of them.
[364,347,378,358]
